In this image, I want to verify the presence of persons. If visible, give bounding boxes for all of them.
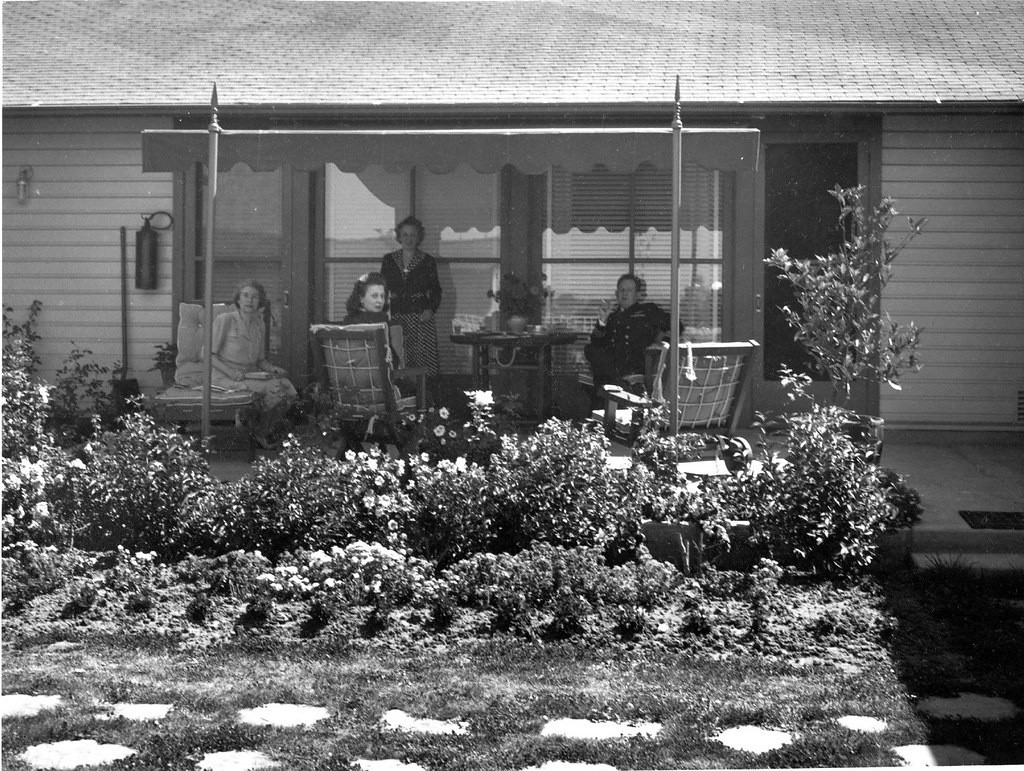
[198,278,299,450]
[584,275,686,396]
[337,272,401,403]
[381,215,443,395]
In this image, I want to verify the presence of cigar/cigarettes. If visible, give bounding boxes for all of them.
[601,299,606,303]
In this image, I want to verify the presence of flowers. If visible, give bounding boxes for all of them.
[485,268,555,318]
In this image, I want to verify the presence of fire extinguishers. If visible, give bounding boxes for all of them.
[134,211,174,290]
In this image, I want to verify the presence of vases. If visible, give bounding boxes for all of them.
[505,313,526,336]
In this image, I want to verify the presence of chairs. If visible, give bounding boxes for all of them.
[308,322,429,462]
[591,338,760,477]
[155,301,270,463]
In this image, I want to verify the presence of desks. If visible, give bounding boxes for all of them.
[448,329,580,425]
